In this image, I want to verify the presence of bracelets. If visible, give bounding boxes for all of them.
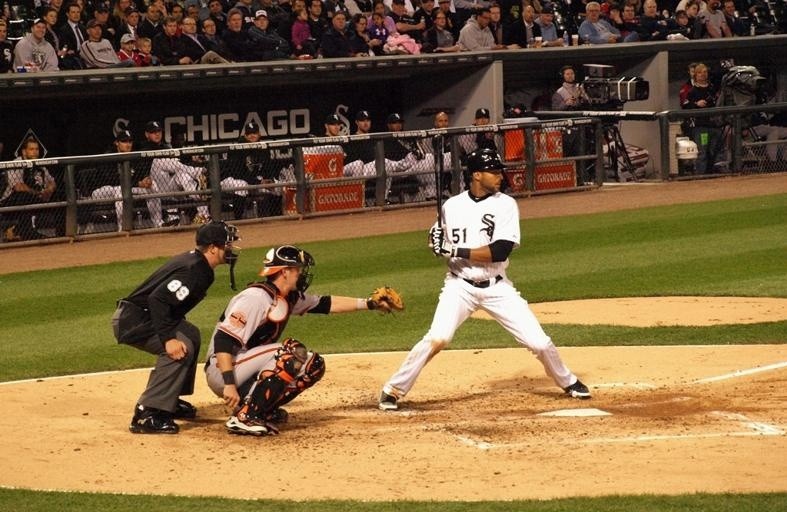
[223,371,235,384]
[358,299,369,310]
[457,248,470,259]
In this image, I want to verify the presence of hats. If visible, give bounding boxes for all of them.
[324,113,342,125]
[145,121,163,131]
[387,113,403,123]
[30,0,555,44]
[117,130,134,142]
[244,123,259,134]
[475,108,490,118]
[354,111,370,120]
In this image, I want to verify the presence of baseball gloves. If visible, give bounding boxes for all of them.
[367,284,405,313]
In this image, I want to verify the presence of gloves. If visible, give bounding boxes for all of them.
[430,228,458,258]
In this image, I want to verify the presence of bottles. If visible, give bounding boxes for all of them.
[562,30,569,47]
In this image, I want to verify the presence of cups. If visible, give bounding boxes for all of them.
[571,35,578,47]
[534,37,542,48]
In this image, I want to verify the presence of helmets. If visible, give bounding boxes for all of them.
[196,221,241,267]
[466,148,508,173]
[257,244,316,293]
[509,104,528,118]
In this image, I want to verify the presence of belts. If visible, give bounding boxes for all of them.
[450,271,503,288]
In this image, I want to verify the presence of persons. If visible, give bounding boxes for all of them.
[228,123,281,217]
[679,63,787,174]
[379,146,593,411]
[111,219,242,434]
[203,244,404,437]
[2,141,65,240]
[552,66,590,184]
[85,122,247,229]
[1,0,787,73]
[321,109,511,206]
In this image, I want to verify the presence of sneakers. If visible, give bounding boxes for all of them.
[565,379,591,400]
[12,213,209,242]
[264,407,290,423]
[224,412,281,438]
[378,390,399,412]
[130,406,179,435]
[174,399,195,419]
